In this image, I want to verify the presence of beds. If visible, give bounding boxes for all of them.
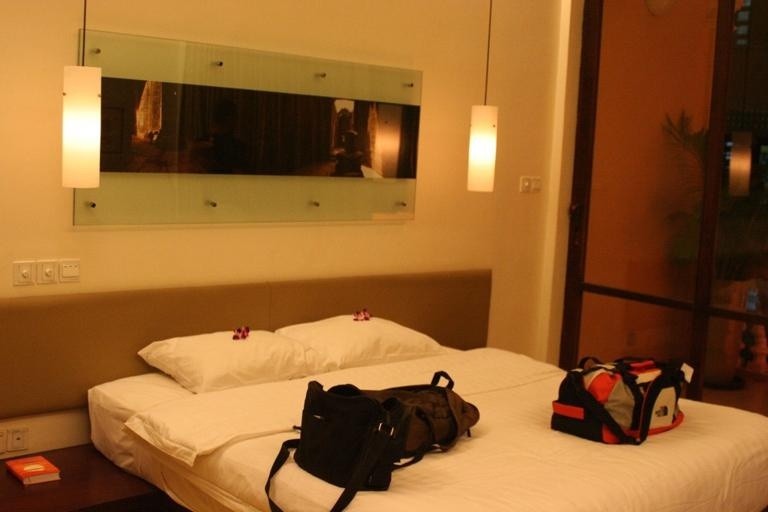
[88,308,768,512]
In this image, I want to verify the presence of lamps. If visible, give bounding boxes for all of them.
[62,0,101,189]
[467,0,500,193]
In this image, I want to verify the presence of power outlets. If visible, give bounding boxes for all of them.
[0,430,7,454]
[7,426,31,452]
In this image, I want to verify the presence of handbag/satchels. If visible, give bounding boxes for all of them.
[552,357,685,445]
[293,371,480,491]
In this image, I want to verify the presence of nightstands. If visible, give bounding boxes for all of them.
[0,442,160,512]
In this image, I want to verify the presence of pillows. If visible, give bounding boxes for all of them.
[273,308,452,369]
[137,326,340,395]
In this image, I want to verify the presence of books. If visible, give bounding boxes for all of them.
[4,455,61,486]
